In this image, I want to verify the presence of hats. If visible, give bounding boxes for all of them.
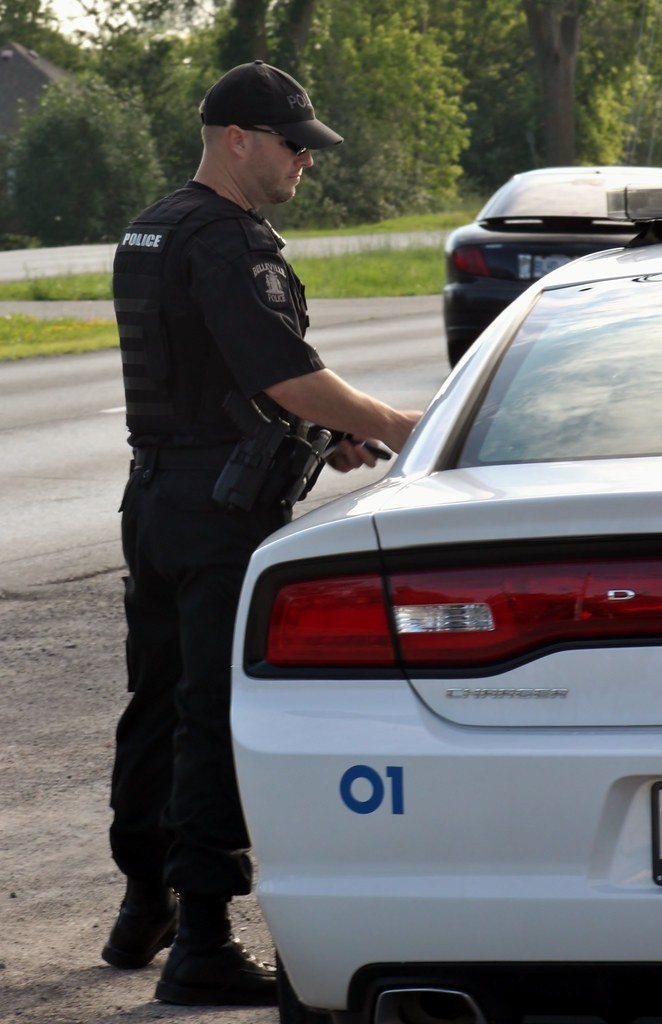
[200,59,344,150]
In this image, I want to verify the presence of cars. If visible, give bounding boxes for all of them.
[443,167,662,369]
[231,190,662,1024]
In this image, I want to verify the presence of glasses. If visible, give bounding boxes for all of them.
[240,124,308,155]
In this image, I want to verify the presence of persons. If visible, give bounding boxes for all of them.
[101,61,425,1007]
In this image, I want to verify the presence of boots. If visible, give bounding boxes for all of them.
[155,899,281,1007]
[102,876,180,970]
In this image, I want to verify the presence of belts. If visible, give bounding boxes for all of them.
[134,444,237,471]
